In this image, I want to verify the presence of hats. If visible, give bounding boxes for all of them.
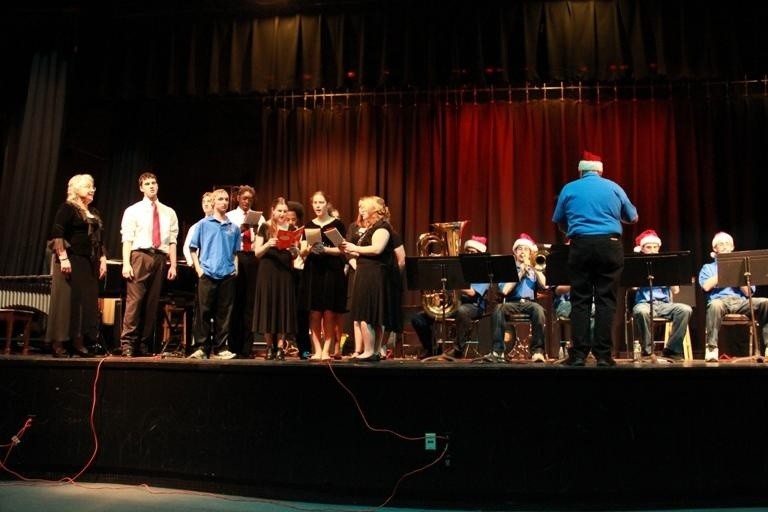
[464,235,487,253]
[633,229,661,253]
[512,233,539,256]
[578,150,604,173]
[711,232,734,247]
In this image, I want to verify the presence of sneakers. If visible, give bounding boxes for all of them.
[704,346,719,361]
[189,349,208,359]
[214,350,237,360]
[531,353,545,362]
[484,351,504,362]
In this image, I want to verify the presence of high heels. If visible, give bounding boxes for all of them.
[275,347,284,361]
[265,345,274,360]
[51,343,67,358]
[70,346,96,357]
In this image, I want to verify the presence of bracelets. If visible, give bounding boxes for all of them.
[58,258,69,261]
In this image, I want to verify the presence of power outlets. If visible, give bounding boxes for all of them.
[424,433,437,453]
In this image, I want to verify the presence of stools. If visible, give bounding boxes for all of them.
[392,305,428,352]
[161,298,189,356]
[716,313,759,355]
[1,305,38,359]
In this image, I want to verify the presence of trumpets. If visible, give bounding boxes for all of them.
[520,250,550,271]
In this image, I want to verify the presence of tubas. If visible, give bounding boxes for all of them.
[416,221,472,318]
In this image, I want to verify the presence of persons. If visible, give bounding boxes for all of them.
[483,229,549,363]
[119,172,180,357]
[411,234,488,358]
[557,231,601,358]
[183,184,410,361]
[631,228,695,359]
[697,230,768,362]
[551,151,639,367]
[46,173,111,357]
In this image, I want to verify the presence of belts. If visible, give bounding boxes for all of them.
[146,248,156,254]
[518,298,526,303]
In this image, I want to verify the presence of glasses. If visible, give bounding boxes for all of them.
[86,185,97,192]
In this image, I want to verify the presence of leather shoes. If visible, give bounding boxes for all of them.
[308,352,387,361]
[569,357,586,365]
[597,358,611,366]
[122,349,133,356]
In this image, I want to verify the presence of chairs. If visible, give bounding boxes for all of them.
[554,280,593,360]
[432,283,488,355]
[623,284,692,362]
[492,275,548,360]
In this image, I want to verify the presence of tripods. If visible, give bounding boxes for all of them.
[732,274,768,364]
[469,274,512,365]
[420,278,457,363]
[628,275,674,364]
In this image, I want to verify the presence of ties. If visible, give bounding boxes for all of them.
[243,212,251,254]
[151,203,161,249]
